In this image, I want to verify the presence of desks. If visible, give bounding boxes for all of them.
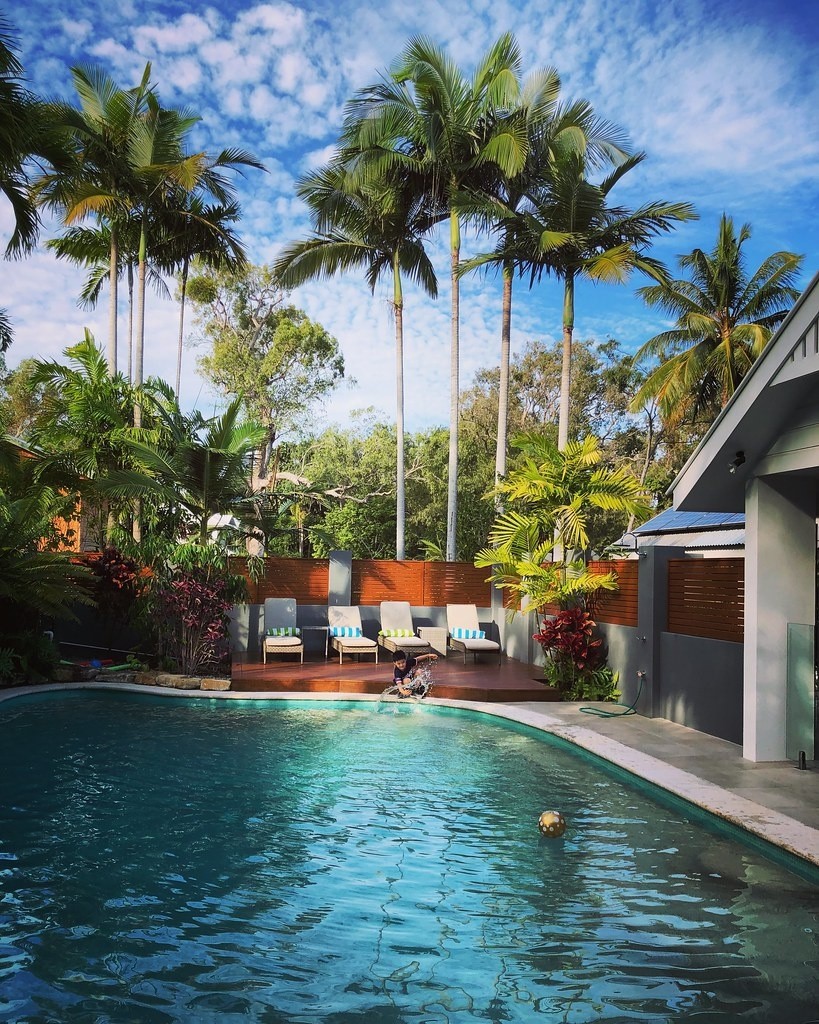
[417,626,448,660]
[301,625,329,659]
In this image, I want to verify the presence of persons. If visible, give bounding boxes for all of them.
[392,650,438,700]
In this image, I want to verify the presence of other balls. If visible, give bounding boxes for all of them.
[539,809,566,837]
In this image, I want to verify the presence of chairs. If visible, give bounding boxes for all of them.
[376,601,431,665]
[262,598,304,665]
[326,605,379,666]
[446,604,502,667]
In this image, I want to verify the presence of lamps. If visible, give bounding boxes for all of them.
[613,532,648,558]
[727,451,745,474]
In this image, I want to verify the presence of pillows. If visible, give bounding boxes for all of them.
[267,627,301,637]
[451,628,486,639]
[378,629,413,637]
[327,626,362,637]
